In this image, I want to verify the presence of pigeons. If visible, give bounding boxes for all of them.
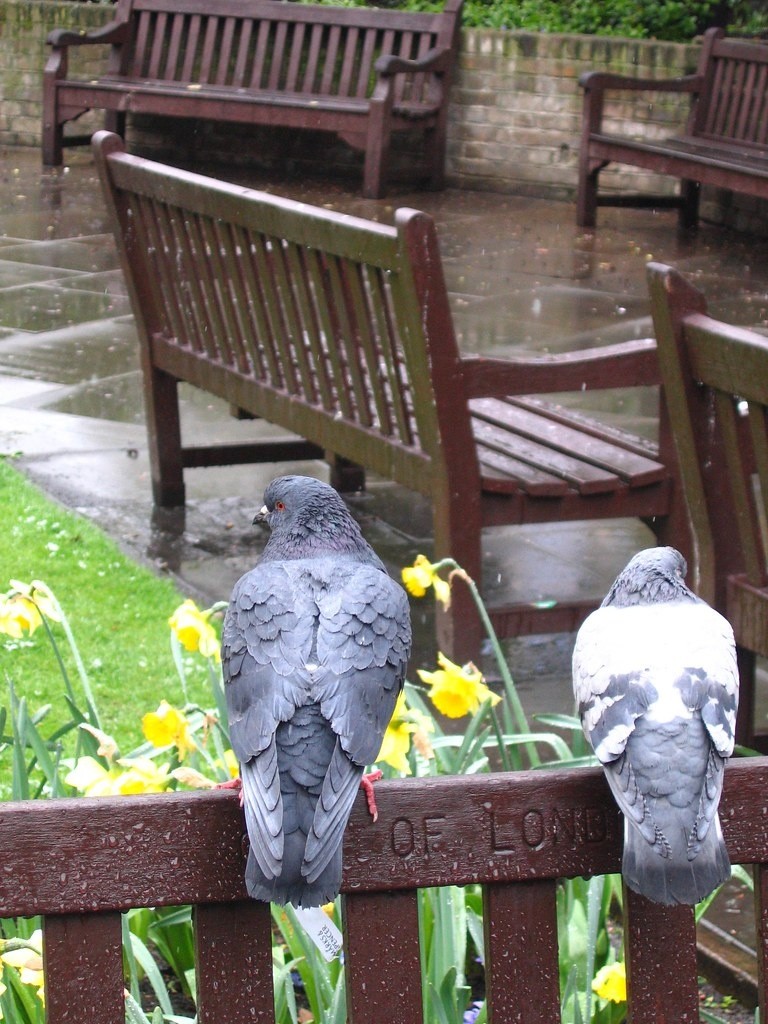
[570,546,742,909]
[218,473,415,911]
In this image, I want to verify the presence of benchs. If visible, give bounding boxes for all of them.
[643,256,768,753]
[576,27,768,247]
[86,127,685,684]
[39,0,465,199]
